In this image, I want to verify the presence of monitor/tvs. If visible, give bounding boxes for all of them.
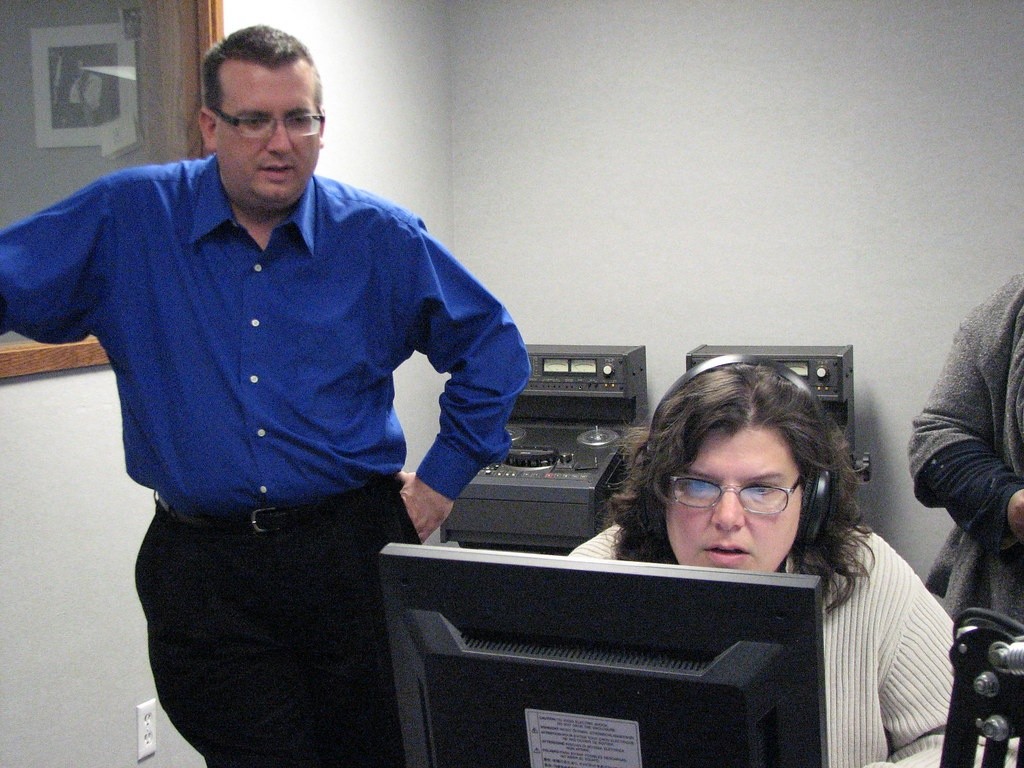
[380,543,830,768]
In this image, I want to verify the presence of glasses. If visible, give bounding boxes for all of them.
[669,473,808,515]
[210,106,327,141]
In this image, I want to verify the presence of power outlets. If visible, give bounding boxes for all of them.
[136,698,156,760]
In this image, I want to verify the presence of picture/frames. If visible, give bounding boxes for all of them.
[29,22,139,148]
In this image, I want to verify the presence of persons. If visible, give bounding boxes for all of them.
[0,25,531,768]
[907,274,1024,624]
[568,355,1019,768]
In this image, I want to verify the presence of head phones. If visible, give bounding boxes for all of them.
[643,353,838,546]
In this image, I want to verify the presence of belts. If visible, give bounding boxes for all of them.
[156,494,331,532]
